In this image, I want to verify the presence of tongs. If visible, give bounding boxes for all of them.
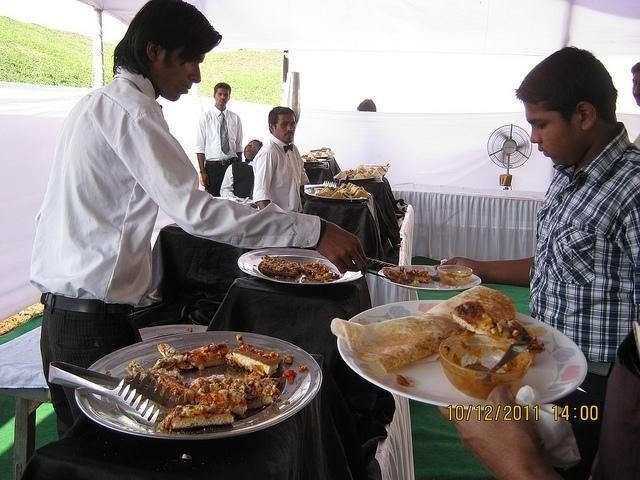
[47,358,164,426]
[354,255,397,279]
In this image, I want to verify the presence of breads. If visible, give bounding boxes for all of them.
[157,343,281,430]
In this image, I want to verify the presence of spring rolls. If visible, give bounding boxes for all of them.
[329,285,515,373]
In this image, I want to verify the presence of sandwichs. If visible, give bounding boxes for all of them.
[317,182,369,198]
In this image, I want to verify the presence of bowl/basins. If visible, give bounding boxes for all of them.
[437,265,473,286]
[438,330,532,401]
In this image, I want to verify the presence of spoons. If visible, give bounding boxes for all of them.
[463,341,531,380]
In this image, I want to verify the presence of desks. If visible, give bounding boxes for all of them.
[386,184,544,285]
[18,150,414,479]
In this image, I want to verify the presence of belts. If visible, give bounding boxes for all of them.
[206,157,237,166]
[44,294,133,317]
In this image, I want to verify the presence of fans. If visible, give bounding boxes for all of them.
[486,121,534,189]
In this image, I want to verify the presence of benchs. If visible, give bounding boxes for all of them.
[0,322,55,480]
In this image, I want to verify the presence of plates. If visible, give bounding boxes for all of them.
[237,248,364,285]
[379,263,482,291]
[300,149,334,165]
[335,299,588,412]
[303,184,371,201]
[335,170,386,181]
[138,323,208,341]
[75,331,324,440]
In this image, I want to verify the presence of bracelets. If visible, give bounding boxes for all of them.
[199,170,206,174]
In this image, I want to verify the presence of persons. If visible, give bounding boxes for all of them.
[438,47,640,480]
[251,106,312,214]
[629,62,640,154]
[194,82,244,191]
[220,138,263,205]
[438,317,639,480]
[38,1,372,444]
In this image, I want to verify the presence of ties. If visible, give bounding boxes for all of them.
[219,112,230,155]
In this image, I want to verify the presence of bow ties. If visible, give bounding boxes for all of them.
[283,143,294,154]
